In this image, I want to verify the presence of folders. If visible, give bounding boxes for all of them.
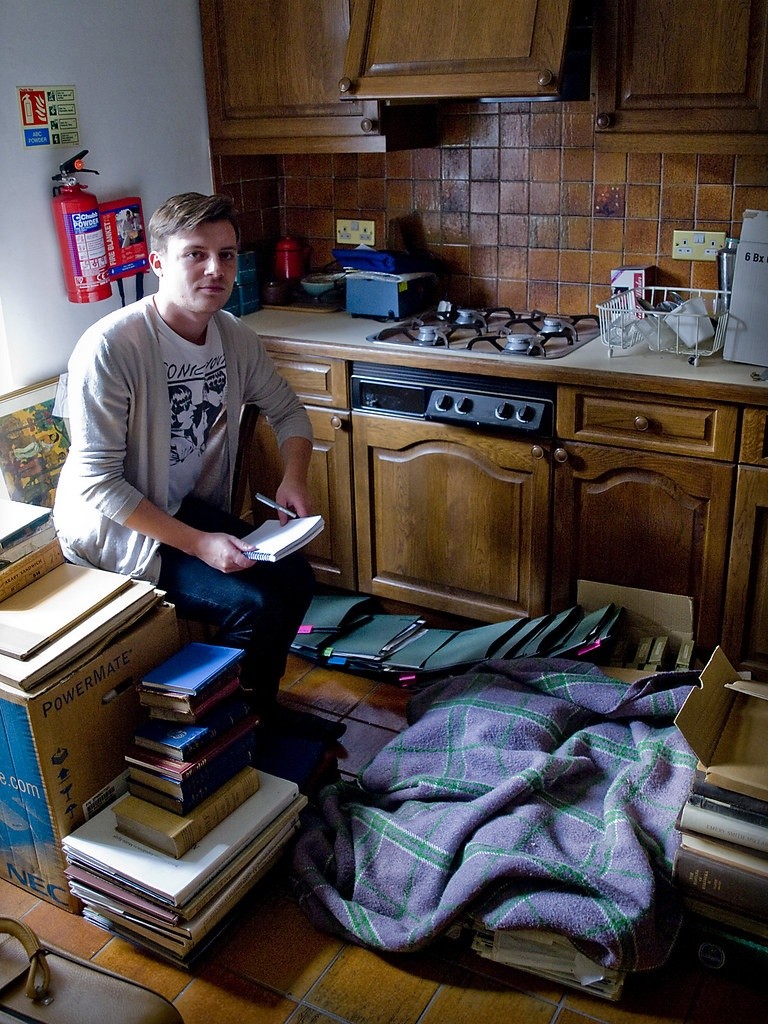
[289,589,622,688]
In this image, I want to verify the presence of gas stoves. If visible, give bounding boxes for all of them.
[348,296,607,435]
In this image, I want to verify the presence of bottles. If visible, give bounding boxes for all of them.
[718,236,739,316]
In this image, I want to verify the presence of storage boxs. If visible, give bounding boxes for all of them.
[722,209,768,367]
[673,646,768,801]
[0,601,178,914]
[577,579,694,684]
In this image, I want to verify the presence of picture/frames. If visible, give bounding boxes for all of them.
[0,375,72,508]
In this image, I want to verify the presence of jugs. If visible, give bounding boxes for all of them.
[276,236,311,284]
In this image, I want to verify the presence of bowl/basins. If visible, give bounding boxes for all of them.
[301,278,334,295]
[260,280,292,306]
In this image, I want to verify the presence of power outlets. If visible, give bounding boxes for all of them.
[672,230,726,262]
[337,219,375,246]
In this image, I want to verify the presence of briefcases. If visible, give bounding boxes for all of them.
[0,916,185,1024]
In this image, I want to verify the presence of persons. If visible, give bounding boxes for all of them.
[51,193,313,755]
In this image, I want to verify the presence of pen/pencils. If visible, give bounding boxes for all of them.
[254,490,298,519]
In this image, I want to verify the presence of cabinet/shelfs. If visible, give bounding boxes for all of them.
[247,352,768,681]
[199,0,768,158]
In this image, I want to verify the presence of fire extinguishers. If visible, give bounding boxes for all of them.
[51,147,114,313]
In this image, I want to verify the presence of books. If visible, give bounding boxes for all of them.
[471,923,628,1001]
[238,515,324,563]
[664,692,768,965]
[0,500,169,692]
[59,641,340,974]
